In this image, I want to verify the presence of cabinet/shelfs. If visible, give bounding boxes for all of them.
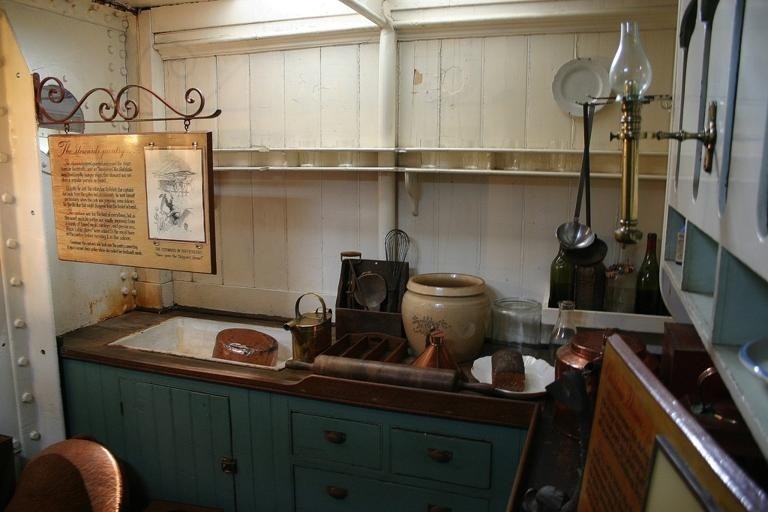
[56,354,526,512]
[661,0,768,466]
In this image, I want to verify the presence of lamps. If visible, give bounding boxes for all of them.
[605,19,718,248]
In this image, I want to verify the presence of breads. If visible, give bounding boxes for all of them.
[491,347,526,392]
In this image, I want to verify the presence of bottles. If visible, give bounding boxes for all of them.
[491,298,542,353]
[550,231,659,434]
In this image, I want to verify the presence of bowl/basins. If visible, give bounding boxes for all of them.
[212,328,280,365]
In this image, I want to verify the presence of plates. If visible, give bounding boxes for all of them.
[470,352,556,399]
[552,59,612,118]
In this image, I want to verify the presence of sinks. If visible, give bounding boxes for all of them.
[104,315,295,371]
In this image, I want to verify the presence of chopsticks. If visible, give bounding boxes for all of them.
[737,334,768,381]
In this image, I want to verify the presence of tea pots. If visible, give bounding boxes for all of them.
[284,293,332,368]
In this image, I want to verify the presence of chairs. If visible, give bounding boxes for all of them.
[4,440,122,512]
